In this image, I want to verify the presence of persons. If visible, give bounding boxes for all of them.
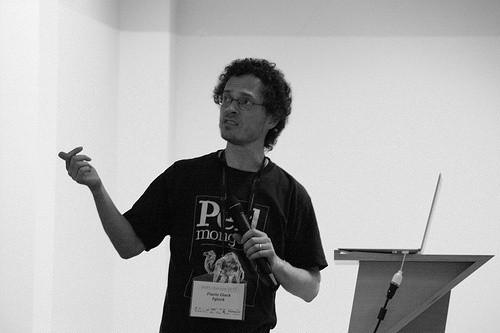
[59,58,328,333]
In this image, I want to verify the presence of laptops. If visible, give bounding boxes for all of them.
[338,172,441,254]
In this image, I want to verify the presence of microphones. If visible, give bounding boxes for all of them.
[225,196,278,289]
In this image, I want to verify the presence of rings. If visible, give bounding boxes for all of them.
[259,244,262,250]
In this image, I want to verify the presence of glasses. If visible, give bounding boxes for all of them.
[219,94,266,111]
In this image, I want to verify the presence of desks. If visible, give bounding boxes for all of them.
[333,249,495,333]
